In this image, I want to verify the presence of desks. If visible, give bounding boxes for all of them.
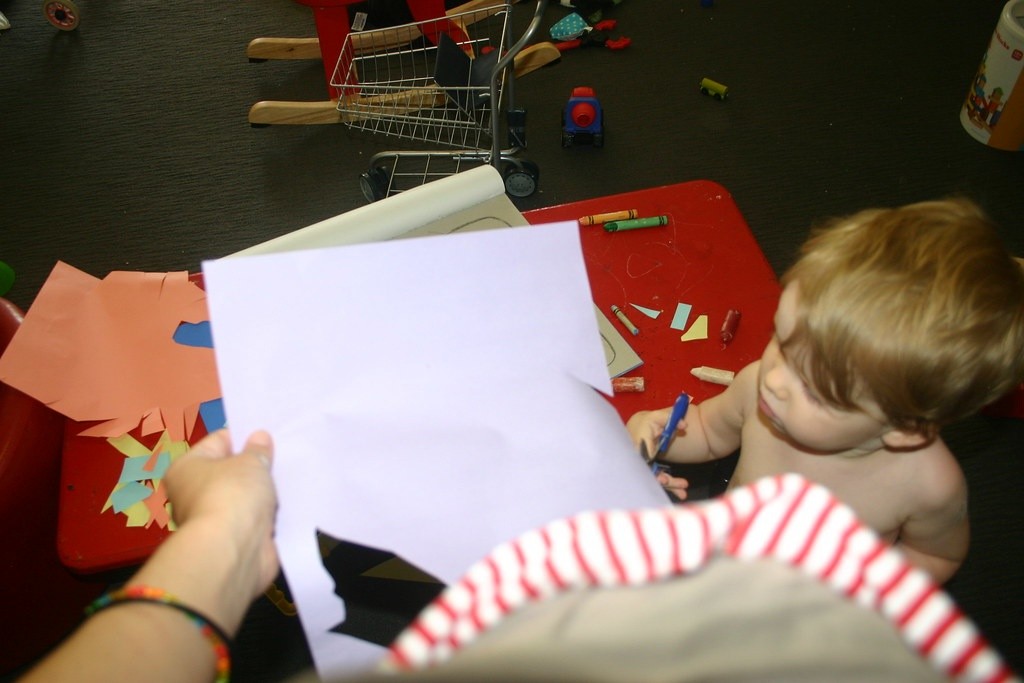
[55,176,784,572]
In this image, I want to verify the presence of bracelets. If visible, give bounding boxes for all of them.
[85,585,232,683]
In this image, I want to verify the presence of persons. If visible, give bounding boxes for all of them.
[627,197,1023,592]
[18,429,1024,683]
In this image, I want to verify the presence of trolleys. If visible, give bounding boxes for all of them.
[330,0,540,200]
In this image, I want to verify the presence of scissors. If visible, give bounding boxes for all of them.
[646,394,689,474]
